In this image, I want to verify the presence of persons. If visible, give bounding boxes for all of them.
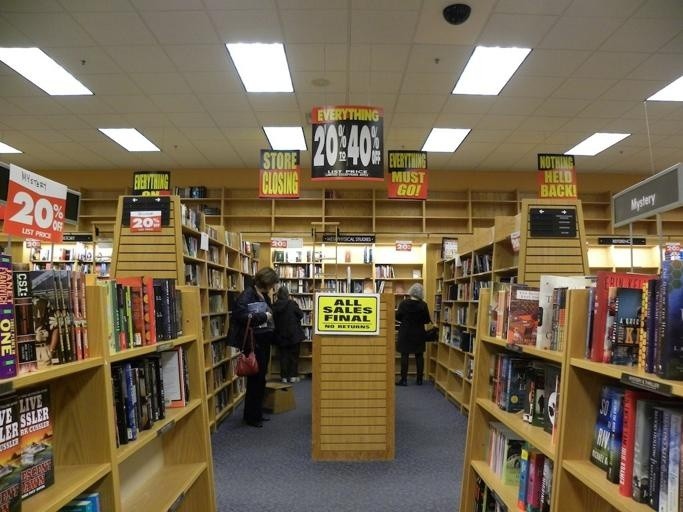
[396,283,431,386]
[271,286,306,383]
[225,267,279,427]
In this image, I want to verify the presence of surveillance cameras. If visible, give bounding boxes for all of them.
[443,4,471,25]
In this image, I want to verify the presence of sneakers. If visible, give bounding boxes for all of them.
[281,377,300,383]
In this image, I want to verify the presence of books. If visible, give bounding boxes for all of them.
[180,204,261,416]
[0,243,111,510]
[471,276,591,512]
[86,274,191,446]
[433,252,493,354]
[272,250,364,342]
[375,265,422,331]
[588,384,682,511]
[585,258,682,386]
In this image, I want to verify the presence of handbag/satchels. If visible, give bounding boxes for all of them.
[424,328,440,342]
[271,320,306,346]
[236,352,259,376]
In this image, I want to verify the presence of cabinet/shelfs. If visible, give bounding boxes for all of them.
[1,190,257,508]
[258,195,419,460]
[420,207,681,511]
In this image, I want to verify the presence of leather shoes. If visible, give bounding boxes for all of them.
[396,378,407,386]
[248,418,271,428]
[417,379,422,385]
